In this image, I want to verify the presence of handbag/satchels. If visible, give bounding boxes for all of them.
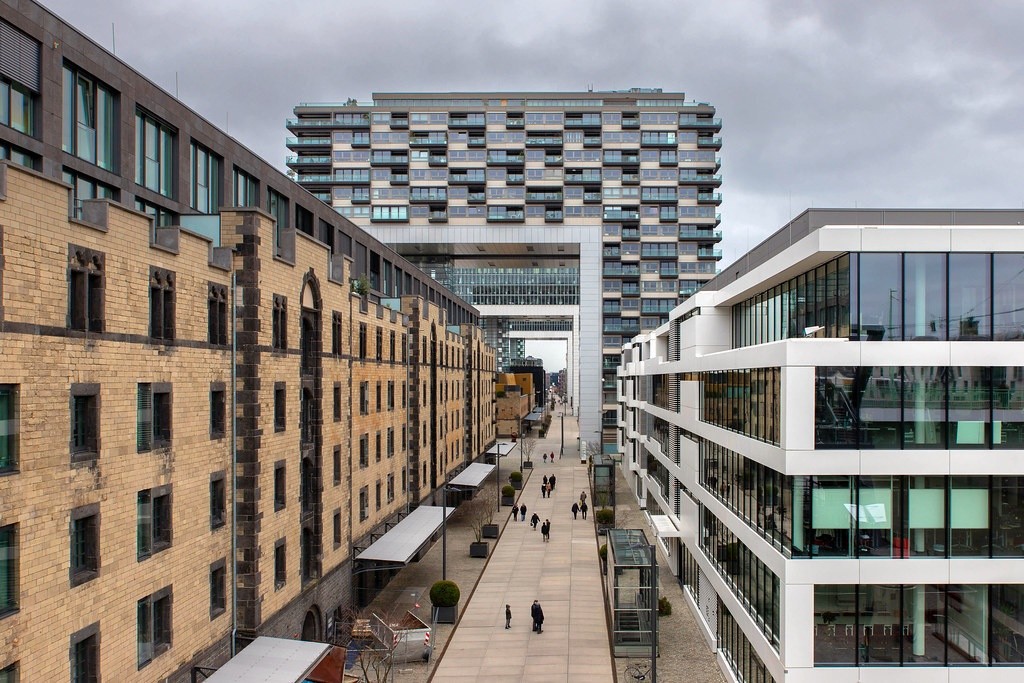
[579,500,581,505]
[530,523,533,526]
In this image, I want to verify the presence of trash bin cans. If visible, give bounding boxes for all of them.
[522,434,525,439]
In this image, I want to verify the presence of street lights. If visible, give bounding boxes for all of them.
[497,443,507,512]
[443,488,461,580]
[521,424,530,473]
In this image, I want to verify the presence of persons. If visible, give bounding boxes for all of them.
[542,519,551,542]
[512,503,519,521]
[541,482,551,498]
[505,604,511,629]
[572,502,579,520]
[719,480,730,499]
[530,512,540,530]
[580,502,588,520]
[548,474,555,490]
[998,381,1012,410]
[511,432,517,442]
[520,503,527,521]
[550,452,554,463]
[531,600,545,634]
[543,452,547,463]
[825,379,836,407]
[580,491,587,504]
[543,475,547,484]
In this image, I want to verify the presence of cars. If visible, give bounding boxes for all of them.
[352,619,371,638]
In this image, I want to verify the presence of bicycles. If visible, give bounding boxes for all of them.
[624,652,664,683]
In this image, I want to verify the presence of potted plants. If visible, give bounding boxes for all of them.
[501,486,515,506]
[517,437,536,468]
[471,486,500,538]
[457,498,491,558]
[509,471,523,489]
[599,543,607,575]
[595,509,615,535]
[766,514,775,529]
[733,472,754,490]
[765,485,778,506]
[658,597,671,617]
[430,580,460,625]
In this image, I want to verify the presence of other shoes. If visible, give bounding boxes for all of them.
[508,626,511,628]
[505,627,509,629]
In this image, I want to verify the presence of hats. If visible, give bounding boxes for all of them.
[535,602,539,606]
